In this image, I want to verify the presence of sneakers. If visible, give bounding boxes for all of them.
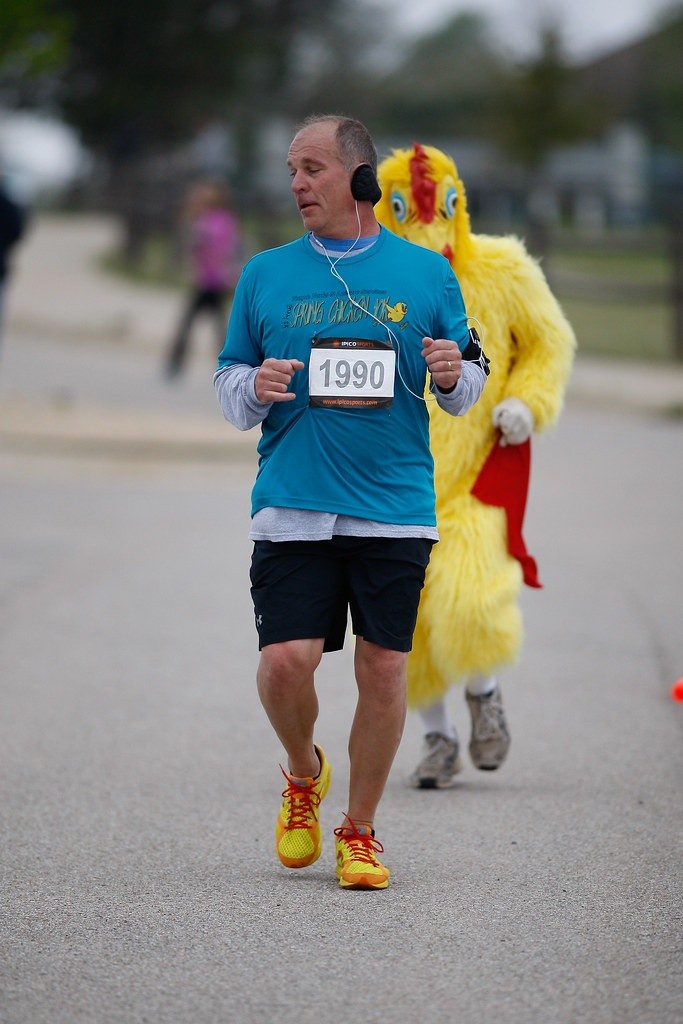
[416,726,463,788]
[466,686,509,772]
[333,812,389,889]
[277,743,332,869]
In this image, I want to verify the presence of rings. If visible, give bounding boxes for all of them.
[448,361,451,370]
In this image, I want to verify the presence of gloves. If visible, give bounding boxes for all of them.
[493,398,534,446]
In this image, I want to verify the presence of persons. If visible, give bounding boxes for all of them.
[159,178,241,376]
[215,114,491,889]
[0,187,26,284]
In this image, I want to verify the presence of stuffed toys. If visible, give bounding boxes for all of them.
[375,144,576,791]
